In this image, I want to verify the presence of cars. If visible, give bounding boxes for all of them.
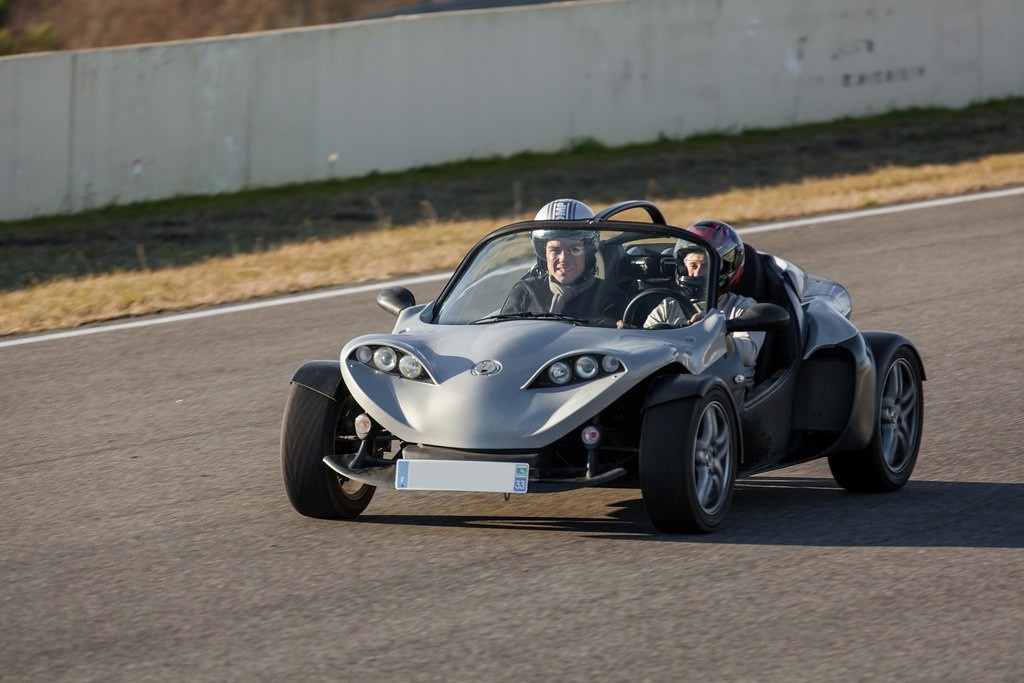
[280,200,931,535]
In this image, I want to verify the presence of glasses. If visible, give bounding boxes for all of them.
[548,246,584,256]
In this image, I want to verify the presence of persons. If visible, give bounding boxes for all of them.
[617,221,764,395]
[500,200,630,328]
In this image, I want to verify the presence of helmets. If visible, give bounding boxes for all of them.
[530,199,599,275]
[673,220,745,301]
